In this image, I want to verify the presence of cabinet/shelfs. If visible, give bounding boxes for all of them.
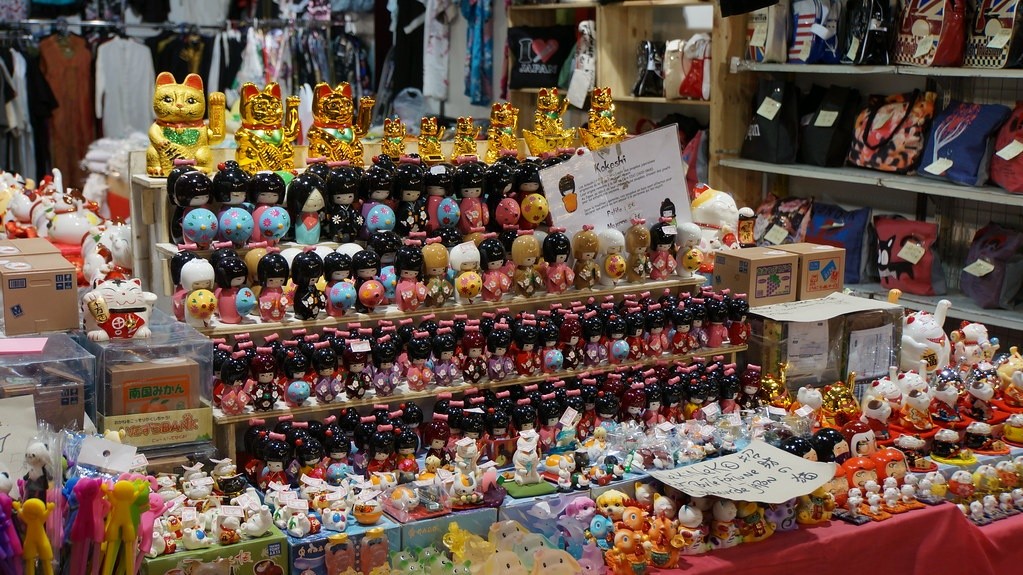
[717,57,1023,333]
[501,0,763,211]
[78,129,1023,575]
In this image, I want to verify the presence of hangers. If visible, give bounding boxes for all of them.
[0,17,358,50]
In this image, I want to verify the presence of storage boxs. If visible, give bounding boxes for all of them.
[136,522,287,575]
[497,458,591,536]
[95,397,212,450]
[0,238,79,335]
[768,243,846,301]
[106,356,199,415]
[712,246,801,308]
[283,511,400,575]
[585,467,650,501]
[0,359,85,432]
[382,507,497,551]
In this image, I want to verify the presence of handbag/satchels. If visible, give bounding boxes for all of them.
[838,0,890,65]
[632,40,666,95]
[799,201,872,283]
[744,0,789,64]
[558,19,598,89]
[754,196,815,249]
[917,100,1012,188]
[662,34,711,103]
[990,104,1023,194]
[847,88,937,175]
[740,78,799,165]
[960,221,1023,310]
[799,84,861,168]
[963,0,1023,69]
[875,221,947,297]
[506,23,574,89]
[787,0,844,63]
[891,0,966,67]
